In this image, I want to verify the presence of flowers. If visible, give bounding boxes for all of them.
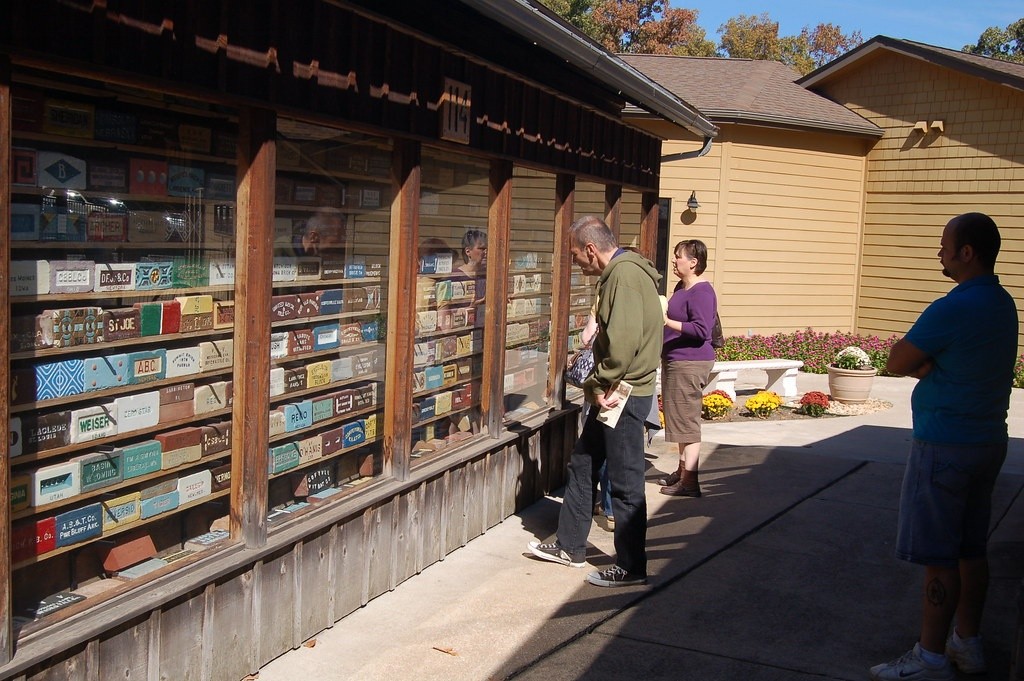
[833,346,872,370]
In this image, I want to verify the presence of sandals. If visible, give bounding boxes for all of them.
[661,481,702,497]
[657,471,683,486]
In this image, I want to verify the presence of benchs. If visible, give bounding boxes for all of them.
[704,358,804,403]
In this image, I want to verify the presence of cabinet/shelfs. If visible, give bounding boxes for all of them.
[12,127,599,639]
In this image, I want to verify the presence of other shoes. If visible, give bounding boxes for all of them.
[594,501,604,515]
[607,516,614,529]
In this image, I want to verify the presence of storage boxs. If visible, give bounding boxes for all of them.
[10,89,588,575]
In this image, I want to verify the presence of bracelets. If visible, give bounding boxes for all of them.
[663,316,668,328]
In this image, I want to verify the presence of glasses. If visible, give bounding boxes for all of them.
[682,239,698,260]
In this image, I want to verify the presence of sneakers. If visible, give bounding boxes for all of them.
[587,564,647,587]
[870,641,957,681]
[527,541,586,568]
[944,636,987,674]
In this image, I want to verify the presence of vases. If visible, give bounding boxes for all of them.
[826,362,878,405]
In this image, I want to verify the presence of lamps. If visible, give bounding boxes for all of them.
[687,189,701,213]
[931,119,944,134]
[913,121,927,136]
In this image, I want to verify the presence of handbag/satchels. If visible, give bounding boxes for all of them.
[564,349,594,388]
[711,313,726,349]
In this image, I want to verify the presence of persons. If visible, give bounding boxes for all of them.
[658,240,717,496]
[871,211,1017,681]
[274,206,345,260]
[582,248,667,530]
[418,230,487,336]
[528,215,664,587]
